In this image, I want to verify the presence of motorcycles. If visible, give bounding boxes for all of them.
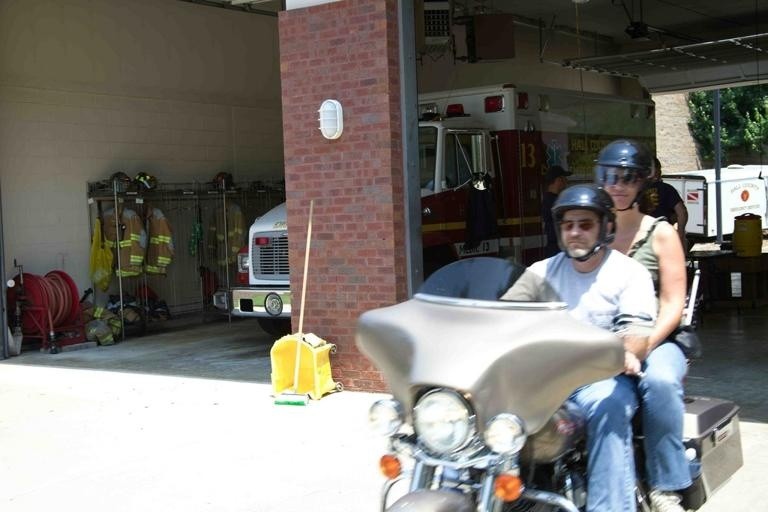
[353,256,741,512]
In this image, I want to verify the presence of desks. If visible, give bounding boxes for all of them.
[690,239,767,313]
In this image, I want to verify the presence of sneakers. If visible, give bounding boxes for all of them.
[648,490,687,512]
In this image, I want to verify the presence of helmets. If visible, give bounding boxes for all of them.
[213,172,233,191]
[111,172,131,193]
[646,157,662,182]
[592,139,647,171]
[550,183,615,224]
[133,172,157,194]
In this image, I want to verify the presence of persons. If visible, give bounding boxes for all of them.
[631,158,688,253]
[499,184,657,512]
[592,140,693,511]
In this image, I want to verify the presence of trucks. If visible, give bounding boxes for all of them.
[212,82,658,338]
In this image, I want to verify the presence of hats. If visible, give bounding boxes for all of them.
[548,166,570,177]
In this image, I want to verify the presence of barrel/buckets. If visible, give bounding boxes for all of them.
[734,213,763,258]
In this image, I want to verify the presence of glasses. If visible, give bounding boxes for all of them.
[555,219,602,231]
[600,173,642,185]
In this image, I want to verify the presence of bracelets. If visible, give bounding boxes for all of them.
[675,223,683,230]
